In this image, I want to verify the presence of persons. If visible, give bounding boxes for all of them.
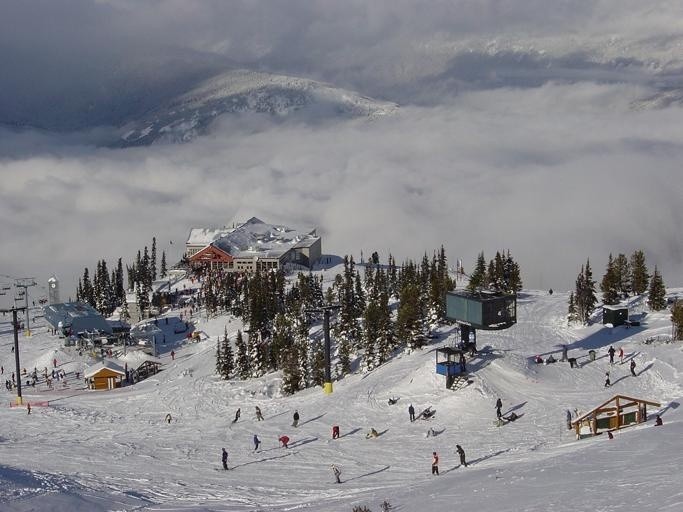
[153,271,204,343]
[630,357,636,376]
[431,451,439,475]
[26,402,31,415]
[533,343,615,369]
[220,447,230,470]
[365,427,378,438]
[253,405,264,421]
[291,409,300,427]
[0,297,113,390]
[231,406,241,422]
[454,444,467,467]
[654,414,662,426]
[332,425,339,439]
[329,462,342,482]
[607,431,614,439]
[407,403,416,422]
[618,347,624,364]
[493,418,504,427]
[496,398,502,409]
[253,433,261,450]
[170,349,175,359]
[603,371,613,388]
[565,408,572,430]
[503,412,517,421]
[496,405,501,418]
[277,435,289,448]
[164,414,171,423]
